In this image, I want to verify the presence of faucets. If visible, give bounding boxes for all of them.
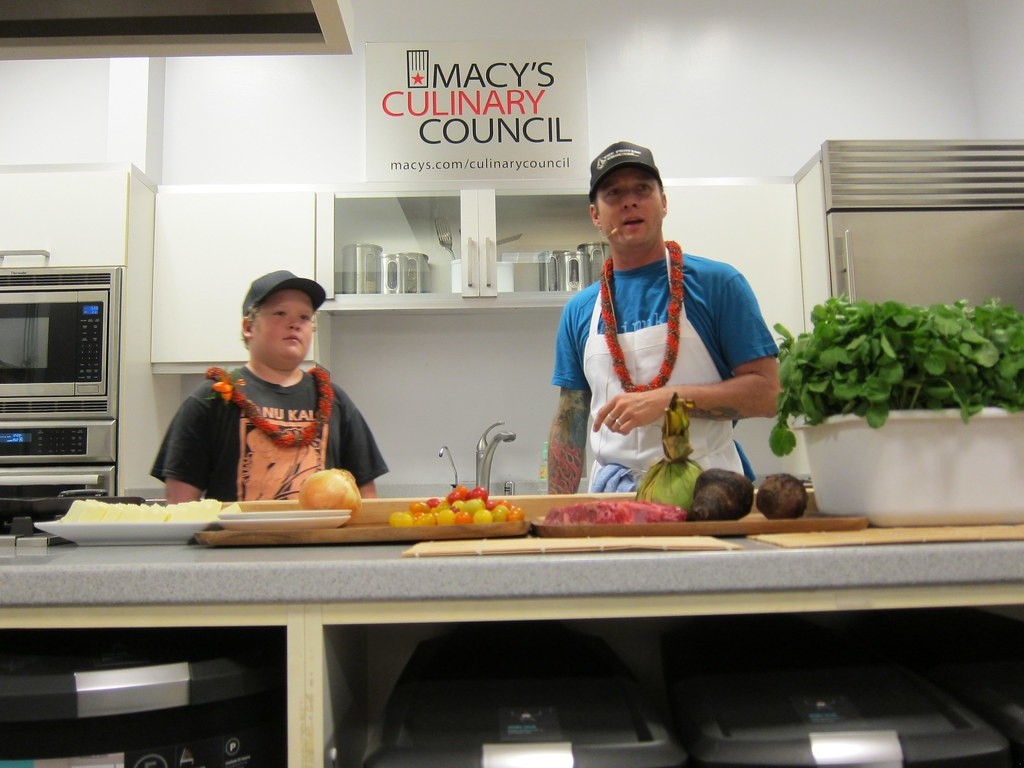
[474,419,518,494]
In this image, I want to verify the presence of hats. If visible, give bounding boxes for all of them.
[589,140,660,202]
[242,271,326,316]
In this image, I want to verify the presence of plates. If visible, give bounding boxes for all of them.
[217,508,354,532]
[33,519,218,548]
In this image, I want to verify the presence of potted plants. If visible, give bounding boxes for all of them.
[767,294,1024,526]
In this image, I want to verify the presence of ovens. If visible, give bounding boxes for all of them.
[0,266,122,535]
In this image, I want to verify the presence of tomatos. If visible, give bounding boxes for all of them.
[389,486,524,527]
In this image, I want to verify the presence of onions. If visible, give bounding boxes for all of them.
[298,467,362,521]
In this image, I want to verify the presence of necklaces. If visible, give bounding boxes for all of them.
[600,239,683,393]
[206,366,333,448]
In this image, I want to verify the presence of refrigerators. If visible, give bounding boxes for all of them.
[795,136,1023,336]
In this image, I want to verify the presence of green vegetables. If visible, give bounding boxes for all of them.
[770,297,1023,458]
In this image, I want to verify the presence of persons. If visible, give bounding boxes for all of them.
[149,270,390,507]
[547,141,780,495]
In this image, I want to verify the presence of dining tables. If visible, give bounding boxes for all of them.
[0,504,1024,768]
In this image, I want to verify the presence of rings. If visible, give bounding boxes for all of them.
[616,418,622,426]
[607,413,616,422]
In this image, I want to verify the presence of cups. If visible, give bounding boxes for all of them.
[342,243,383,295]
[557,251,591,292]
[576,241,612,285]
[378,253,405,295]
[451,259,463,295]
[537,250,572,291]
[404,252,428,294]
